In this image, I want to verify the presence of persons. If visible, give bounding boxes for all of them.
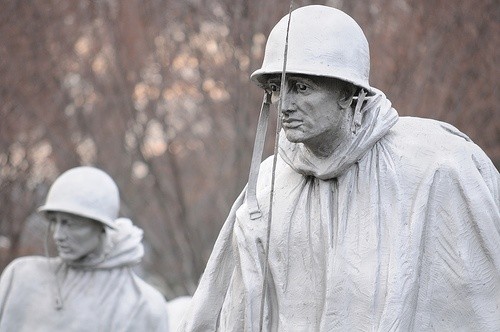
[183,5,500,331]
[0,165,168,332]
